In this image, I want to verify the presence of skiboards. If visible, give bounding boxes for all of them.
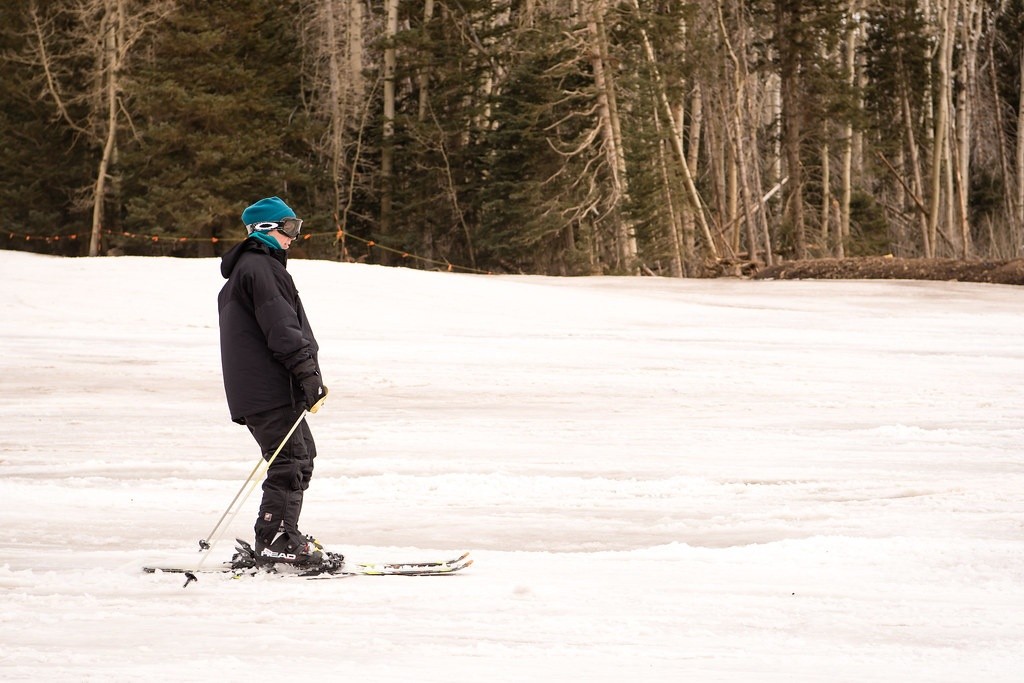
[144,550,477,579]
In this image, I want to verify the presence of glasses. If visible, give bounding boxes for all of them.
[278,217,303,237]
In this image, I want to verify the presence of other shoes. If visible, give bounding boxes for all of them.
[255,533,343,569]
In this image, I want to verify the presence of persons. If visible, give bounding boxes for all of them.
[216,197,330,566]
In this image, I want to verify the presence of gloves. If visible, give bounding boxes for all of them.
[300,373,326,411]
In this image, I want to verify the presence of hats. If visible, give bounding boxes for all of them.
[241,196,295,236]
